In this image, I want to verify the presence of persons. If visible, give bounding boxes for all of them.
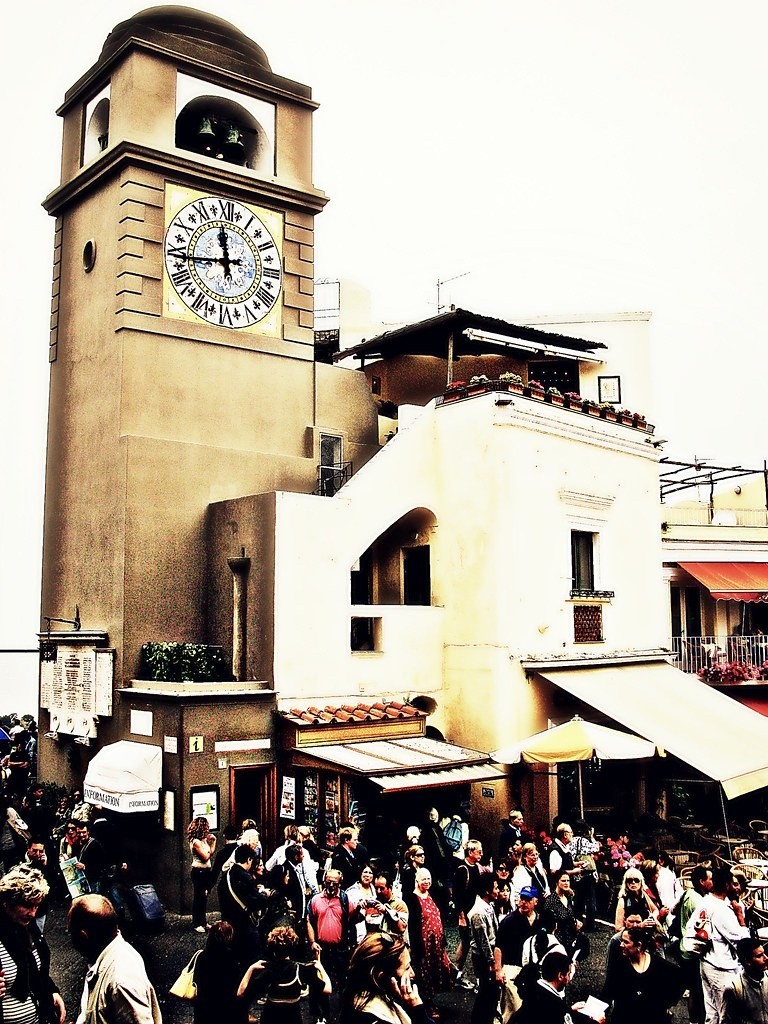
[0,713,768,1024]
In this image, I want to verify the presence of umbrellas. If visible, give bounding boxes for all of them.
[490,715,667,820]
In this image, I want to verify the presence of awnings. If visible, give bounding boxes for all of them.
[677,561,768,604]
[532,659,768,800]
[84,740,163,814]
[368,764,508,792]
